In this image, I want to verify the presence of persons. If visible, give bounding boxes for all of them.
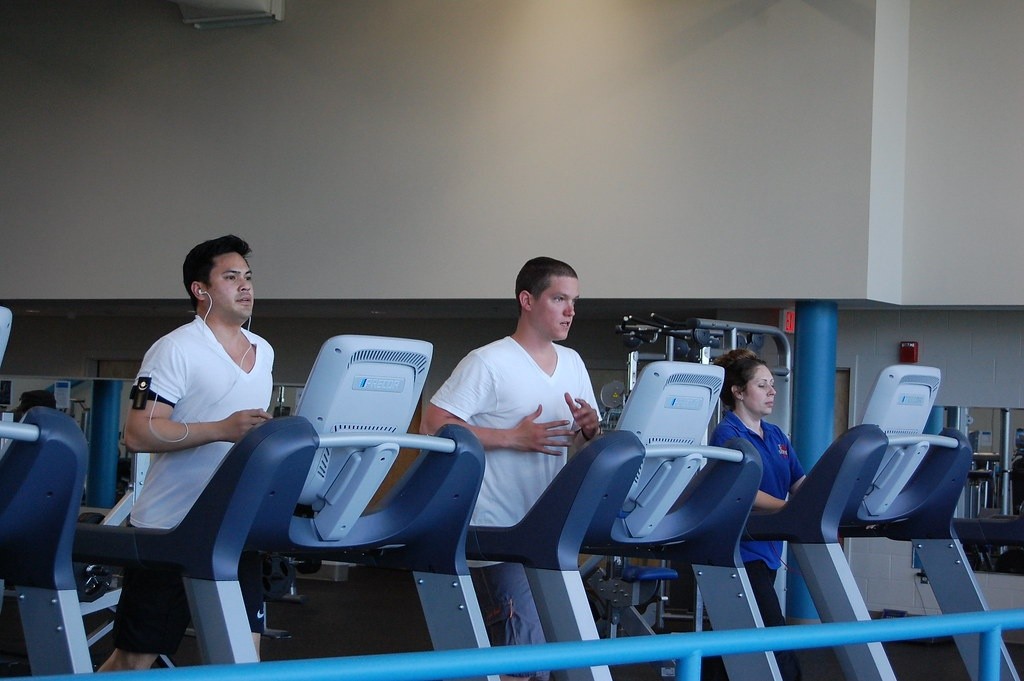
[420,257,601,680]
[7,390,56,422]
[97,234,274,671]
[708,349,806,681]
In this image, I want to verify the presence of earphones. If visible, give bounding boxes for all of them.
[198,289,207,295]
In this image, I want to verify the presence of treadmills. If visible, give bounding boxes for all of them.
[1,307,1024,680]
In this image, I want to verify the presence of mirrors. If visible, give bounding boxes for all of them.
[911,406,1024,576]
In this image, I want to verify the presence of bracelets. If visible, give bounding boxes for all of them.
[581,428,590,441]
